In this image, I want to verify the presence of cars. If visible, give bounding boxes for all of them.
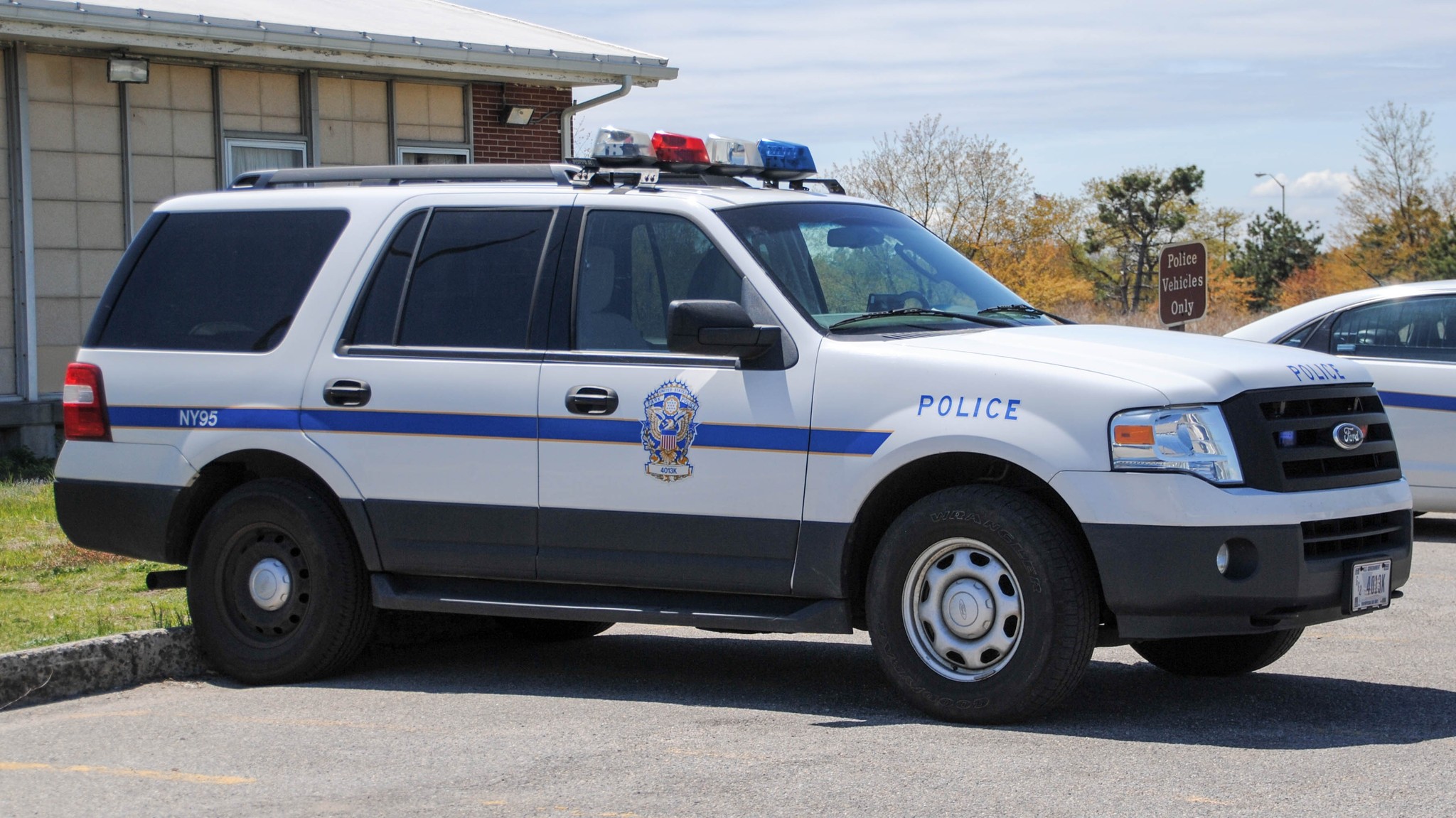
[1219,279,1456,517]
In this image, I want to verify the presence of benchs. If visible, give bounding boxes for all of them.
[1333,329,1400,350]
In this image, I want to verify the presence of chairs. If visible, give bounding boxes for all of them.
[578,247,649,349]
[686,248,742,306]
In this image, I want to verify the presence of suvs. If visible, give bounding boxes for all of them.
[53,129,1414,724]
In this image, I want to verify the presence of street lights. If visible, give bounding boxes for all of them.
[1255,174,1284,219]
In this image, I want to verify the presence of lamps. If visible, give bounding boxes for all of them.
[503,105,536,126]
[107,56,152,84]
